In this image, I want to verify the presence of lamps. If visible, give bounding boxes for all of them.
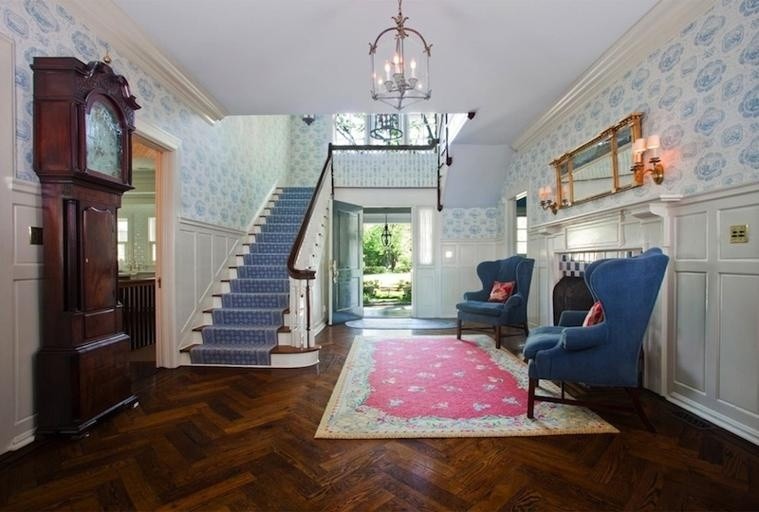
[540,185,555,214]
[630,134,665,187]
[364,1,437,111]
[379,210,395,248]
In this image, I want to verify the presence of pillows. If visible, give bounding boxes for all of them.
[580,298,604,329]
[488,278,517,304]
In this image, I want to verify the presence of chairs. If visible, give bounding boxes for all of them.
[456,253,534,349]
[522,246,672,435]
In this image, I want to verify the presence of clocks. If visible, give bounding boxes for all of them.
[81,93,134,188]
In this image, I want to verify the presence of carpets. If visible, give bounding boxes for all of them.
[314,332,622,442]
[192,187,315,366]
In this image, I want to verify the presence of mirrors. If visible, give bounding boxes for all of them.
[549,111,648,210]
